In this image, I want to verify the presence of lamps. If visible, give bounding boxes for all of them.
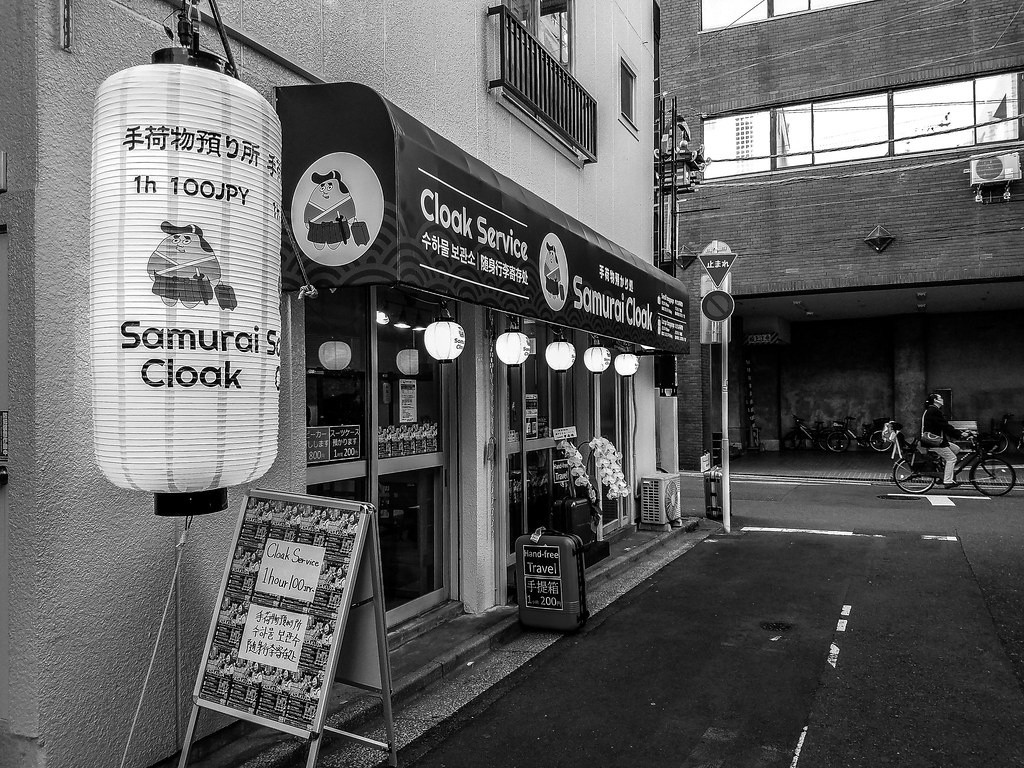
[545,337,577,374]
[423,317,465,363]
[611,351,640,378]
[585,346,614,376]
[496,329,533,368]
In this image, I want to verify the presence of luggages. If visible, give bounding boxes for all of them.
[551,494,593,551]
[559,282,564,302]
[514,529,591,633]
[214,280,237,312]
[702,449,733,522]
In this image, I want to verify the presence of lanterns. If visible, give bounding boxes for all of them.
[583,344,611,375]
[88,48,282,516]
[318,337,351,373]
[424,316,466,363]
[496,328,531,368]
[396,346,419,377]
[545,337,576,373]
[614,351,639,378]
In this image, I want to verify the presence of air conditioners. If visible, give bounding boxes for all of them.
[966,151,1023,187]
[639,473,682,526]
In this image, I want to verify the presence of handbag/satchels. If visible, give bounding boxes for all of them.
[920,431,944,447]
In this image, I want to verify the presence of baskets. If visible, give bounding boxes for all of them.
[833,420,845,431]
[977,435,1001,457]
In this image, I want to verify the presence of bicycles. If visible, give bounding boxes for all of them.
[986,411,1024,454]
[781,413,892,451]
[892,430,1016,497]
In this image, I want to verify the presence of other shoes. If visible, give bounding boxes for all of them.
[943,482,959,488]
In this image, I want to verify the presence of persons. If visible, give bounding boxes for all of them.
[921,393,979,490]
